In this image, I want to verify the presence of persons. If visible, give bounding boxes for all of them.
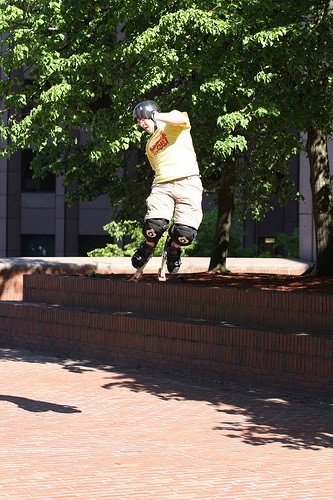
[131,100,205,275]
